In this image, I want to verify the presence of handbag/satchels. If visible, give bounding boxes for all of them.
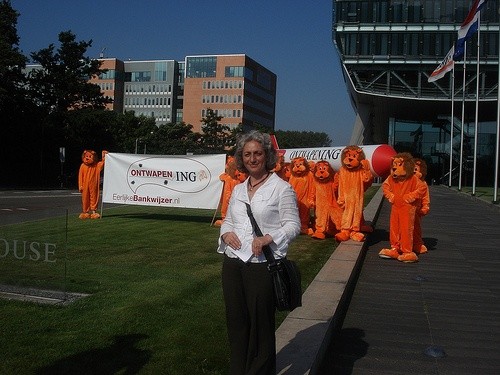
[270,258,303,312]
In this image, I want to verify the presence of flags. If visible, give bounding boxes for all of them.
[453,0,488,61]
[428,46,453,83]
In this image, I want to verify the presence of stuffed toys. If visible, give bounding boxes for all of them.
[214,145,373,242]
[78,150,108,219]
[378,153,431,263]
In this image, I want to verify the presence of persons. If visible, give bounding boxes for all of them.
[216,131,301,375]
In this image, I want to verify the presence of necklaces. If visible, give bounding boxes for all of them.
[248,170,270,191]
